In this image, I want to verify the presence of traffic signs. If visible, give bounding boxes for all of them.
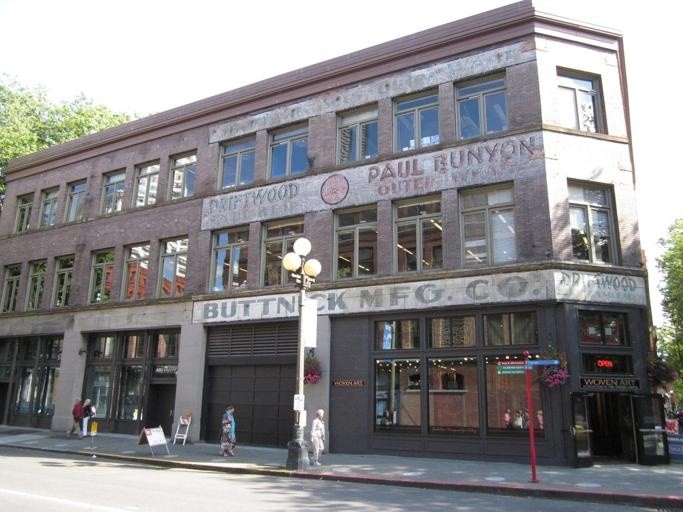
[496,360,559,375]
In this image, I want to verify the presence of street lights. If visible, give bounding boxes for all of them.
[283,237,321,471]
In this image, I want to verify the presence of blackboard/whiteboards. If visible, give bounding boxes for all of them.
[177,424,190,435]
[145,428,167,447]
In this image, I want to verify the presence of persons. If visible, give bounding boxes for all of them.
[80,399,92,437]
[311,409,326,466]
[504,408,544,430]
[382,411,392,425]
[67,398,83,436]
[221,405,237,457]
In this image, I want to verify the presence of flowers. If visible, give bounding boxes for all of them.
[304,355,323,385]
[530,344,572,392]
